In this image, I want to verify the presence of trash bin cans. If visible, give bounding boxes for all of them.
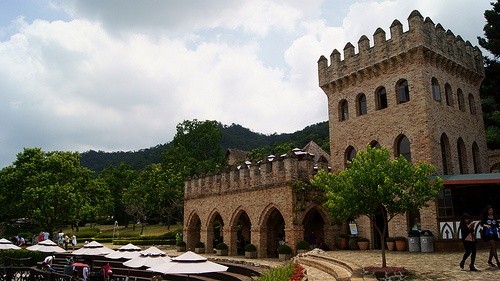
[64,265,72,276]
[408,230,419,251]
[420,230,434,252]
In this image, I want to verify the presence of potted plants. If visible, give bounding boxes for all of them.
[194,242,205,253]
[357,237,369,250]
[217,243,228,256]
[177,241,186,252]
[338,235,347,249]
[277,245,292,259]
[386,236,406,251]
[297,240,309,255]
[245,244,257,258]
[348,238,357,250]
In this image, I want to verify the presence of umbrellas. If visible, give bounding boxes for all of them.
[280,148,315,157]
[257,154,275,163]
[26,239,67,261]
[70,240,116,281]
[105,242,145,281]
[145,250,229,281]
[0,238,23,266]
[237,161,251,170]
[122,246,172,276]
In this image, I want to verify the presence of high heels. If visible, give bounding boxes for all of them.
[497,263,500,268]
[488,261,496,266]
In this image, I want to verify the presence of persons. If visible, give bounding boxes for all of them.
[100,263,113,281]
[31,231,50,245]
[9,236,25,247]
[64,255,94,281]
[40,255,57,281]
[481,206,500,270]
[460,212,478,271]
[53,230,77,250]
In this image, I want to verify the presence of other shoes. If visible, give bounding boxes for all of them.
[460,262,464,269]
[470,267,478,272]
